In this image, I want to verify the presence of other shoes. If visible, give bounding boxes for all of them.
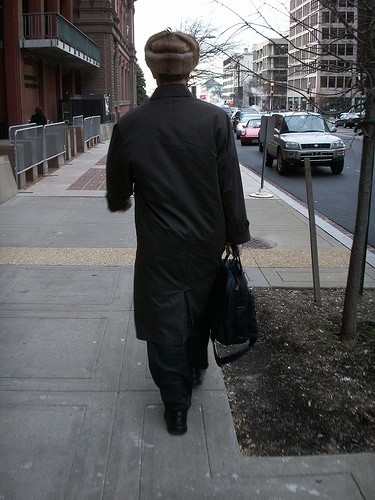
[191,367,206,385]
[164,408,187,435]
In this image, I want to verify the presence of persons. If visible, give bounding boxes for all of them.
[29,107,47,126]
[105,31,251,436]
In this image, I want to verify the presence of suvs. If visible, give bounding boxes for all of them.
[264,111,346,177]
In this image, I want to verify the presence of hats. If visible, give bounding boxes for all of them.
[144,27,200,75]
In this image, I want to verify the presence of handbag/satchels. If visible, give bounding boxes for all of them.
[210,243,258,366]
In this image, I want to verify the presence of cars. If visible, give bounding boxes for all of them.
[335,112,363,129]
[216,103,269,146]
